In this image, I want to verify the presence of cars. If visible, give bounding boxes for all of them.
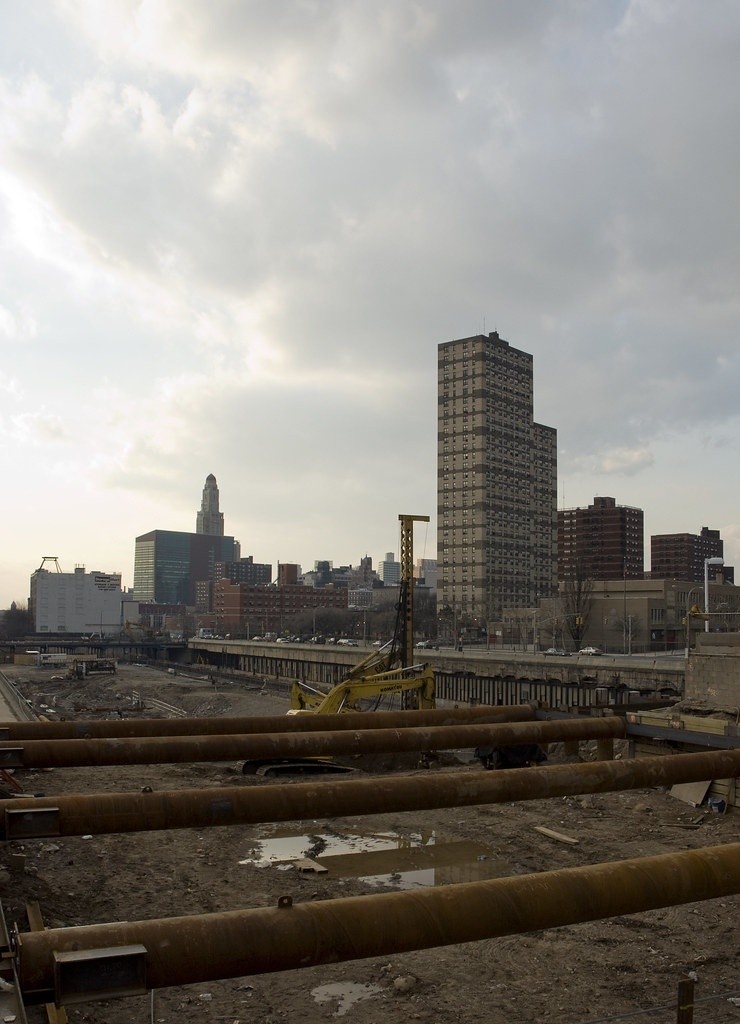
[416,642,432,649]
[253,636,359,647]
[544,648,572,657]
[204,634,235,640]
[579,647,603,656]
[372,640,384,646]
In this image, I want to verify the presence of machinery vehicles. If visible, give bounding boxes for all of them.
[286,515,430,717]
[234,662,439,778]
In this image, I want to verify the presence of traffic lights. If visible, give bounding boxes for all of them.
[576,618,579,624]
[606,618,608,624]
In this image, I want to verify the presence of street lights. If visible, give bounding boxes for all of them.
[686,587,703,651]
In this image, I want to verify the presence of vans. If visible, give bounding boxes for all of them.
[199,628,210,638]
